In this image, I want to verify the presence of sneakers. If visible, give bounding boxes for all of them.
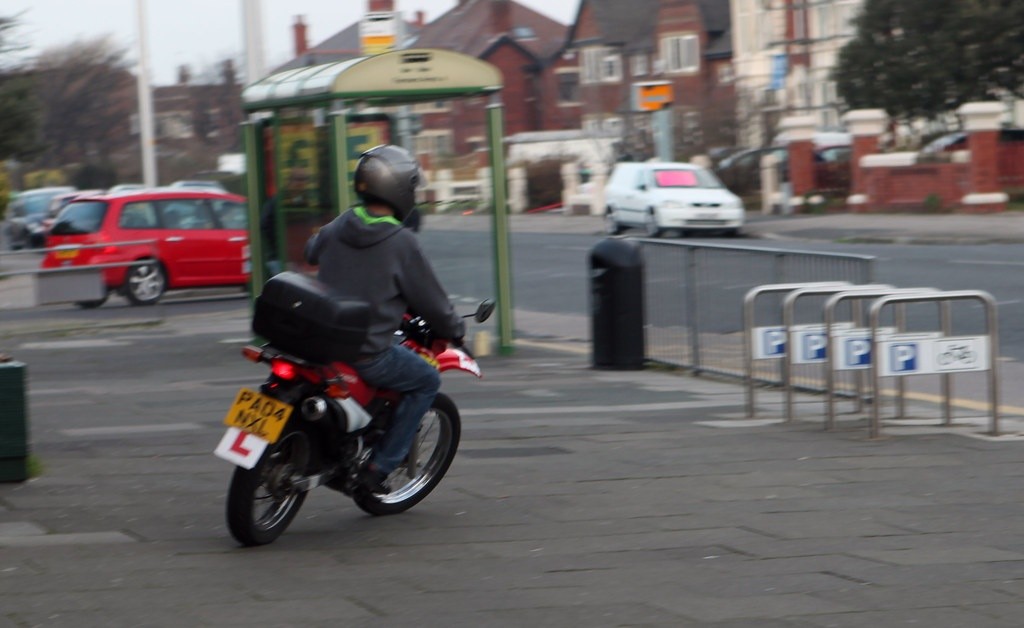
[359,470,392,493]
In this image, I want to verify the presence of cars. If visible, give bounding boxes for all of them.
[45,186,252,305]
[4,172,231,246]
[600,161,746,238]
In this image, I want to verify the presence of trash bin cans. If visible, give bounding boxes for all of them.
[0,356,33,485]
[580,236,651,373]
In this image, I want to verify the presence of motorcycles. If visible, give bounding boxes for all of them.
[215,274,498,547]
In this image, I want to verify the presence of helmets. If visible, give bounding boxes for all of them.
[354,144,420,222]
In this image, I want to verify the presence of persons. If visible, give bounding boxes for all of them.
[303,144,466,495]
